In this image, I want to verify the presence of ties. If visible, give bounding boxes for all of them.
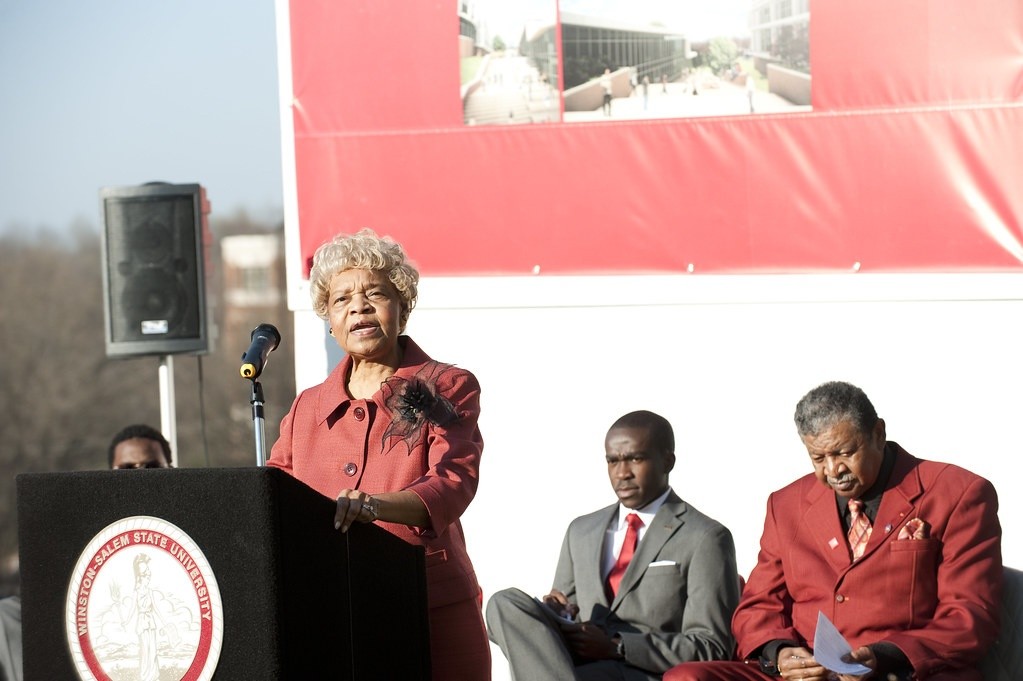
[845,498,873,563]
[604,513,645,610]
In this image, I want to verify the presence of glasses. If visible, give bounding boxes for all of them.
[113,462,167,469]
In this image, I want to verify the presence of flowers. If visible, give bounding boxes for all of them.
[378,359,464,457]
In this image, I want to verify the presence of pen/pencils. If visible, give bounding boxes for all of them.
[561,591,571,621]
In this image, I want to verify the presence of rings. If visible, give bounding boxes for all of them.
[371,511,377,517]
[363,505,373,511]
[576,641,577,649]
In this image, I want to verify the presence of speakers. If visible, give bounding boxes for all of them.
[100,182,212,360]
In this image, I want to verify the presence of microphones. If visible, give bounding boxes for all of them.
[240,324,281,379]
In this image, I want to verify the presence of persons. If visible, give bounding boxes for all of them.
[485,410,741,681]
[629,72,637,95]
[662,378,1003,681]
[108,425,173,470]
[266,227,492,681]
[600,69,612,115]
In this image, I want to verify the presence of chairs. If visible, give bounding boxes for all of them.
[976,567,1023,681]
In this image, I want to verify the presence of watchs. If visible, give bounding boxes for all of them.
[618,640,625,655]
